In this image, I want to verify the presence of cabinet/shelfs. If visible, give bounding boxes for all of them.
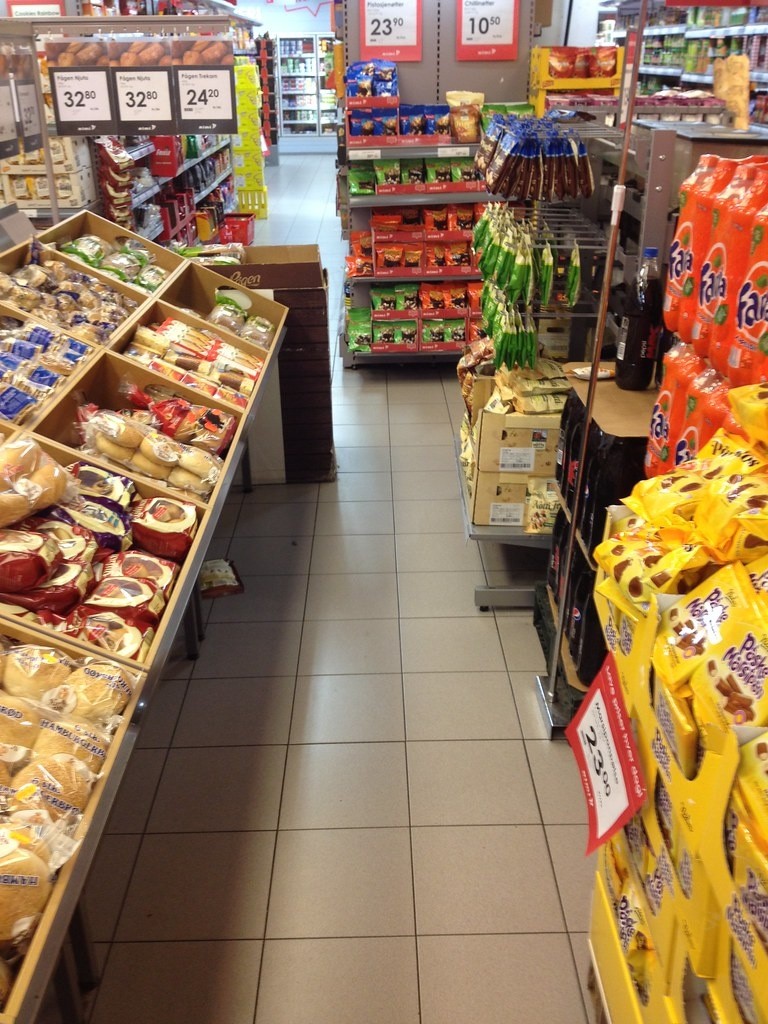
[531,361,661,716]
[256,33,342,166]
[600,1,768,136]
[338,106,512,370]
[0,0,261,249]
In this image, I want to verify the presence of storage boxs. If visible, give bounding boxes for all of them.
[468,375,564,527]
[148,135,256,246]
[0,130,95,209]
[0,208,290,1024]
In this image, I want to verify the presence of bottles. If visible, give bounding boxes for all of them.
[548,508,608,687]
[615,247,664,391]
[556,392,648,566]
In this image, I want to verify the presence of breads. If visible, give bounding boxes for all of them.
[0,234,272,943]
[46,41,234,67]
